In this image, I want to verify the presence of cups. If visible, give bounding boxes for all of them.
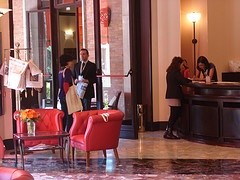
[206,76,210,83]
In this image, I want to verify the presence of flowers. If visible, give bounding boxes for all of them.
[20,109,40,132]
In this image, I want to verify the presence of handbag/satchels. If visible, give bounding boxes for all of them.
[63,68,83,116]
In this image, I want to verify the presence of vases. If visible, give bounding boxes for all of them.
[27,119,35,136]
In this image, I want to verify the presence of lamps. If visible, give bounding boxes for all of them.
[187,12,201,77]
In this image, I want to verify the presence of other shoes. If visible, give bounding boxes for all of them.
[163,129,180,139]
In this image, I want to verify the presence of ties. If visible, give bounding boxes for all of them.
[81,62,85,71]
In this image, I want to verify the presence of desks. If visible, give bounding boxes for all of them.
[13,131,70,169]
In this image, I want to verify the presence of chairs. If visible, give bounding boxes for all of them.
[0,137,34,180]
[222,72,240,82]
[14,109,70,163]
[70,109,124,173]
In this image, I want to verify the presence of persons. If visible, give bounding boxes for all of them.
[58,53,78,136]
[191,55,218,85]
[182,59,191,78]
[71,49,97,111]
[163,56,199,139]
